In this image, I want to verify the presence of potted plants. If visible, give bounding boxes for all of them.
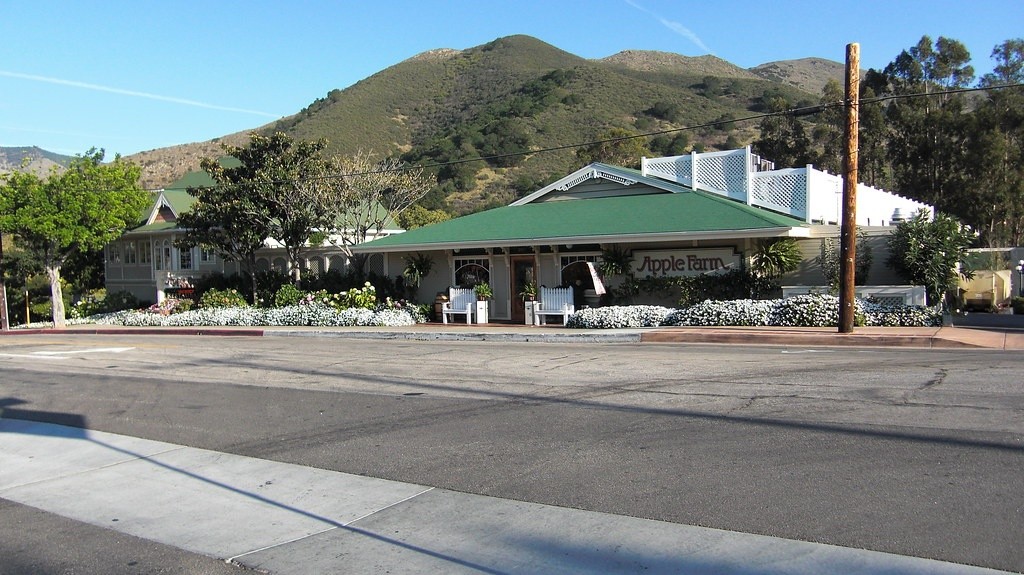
[518,281,537,302]
[473,282,493,301]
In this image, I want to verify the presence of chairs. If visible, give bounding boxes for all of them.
[442,286,477,324]
[532,286,576,326]
